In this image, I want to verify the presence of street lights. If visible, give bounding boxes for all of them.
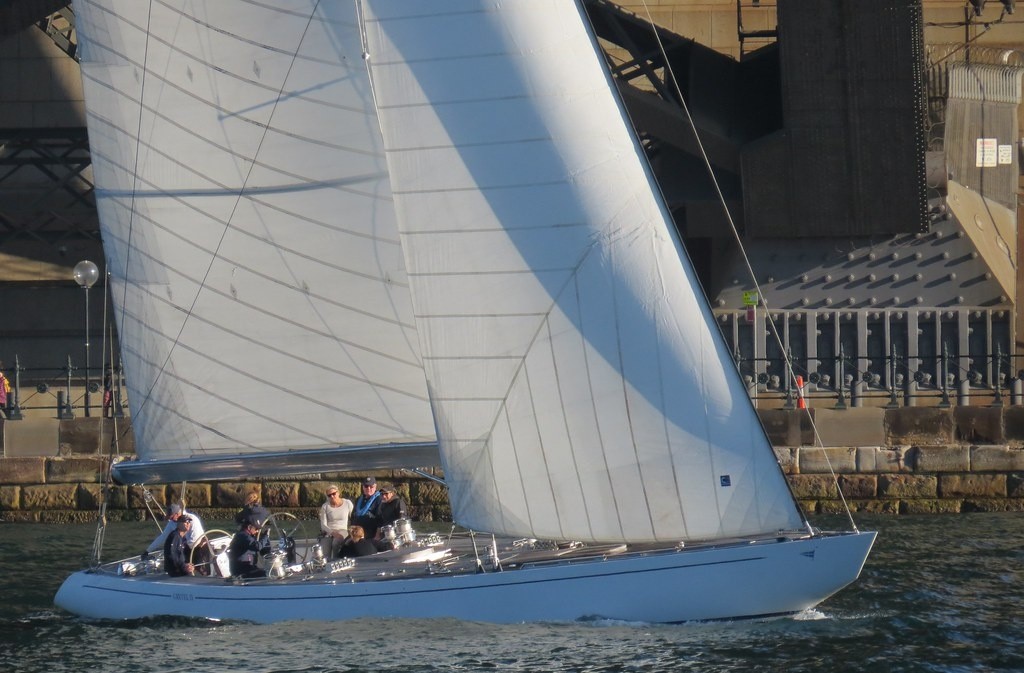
[73,259,99,416]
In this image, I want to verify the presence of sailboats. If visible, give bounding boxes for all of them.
[52,0,879,629]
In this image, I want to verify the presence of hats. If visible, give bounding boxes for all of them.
[177,514,193,522]
[379,484,395,493]
[165,504,180,514]
[362,475,376,485]
[245,517,266,529]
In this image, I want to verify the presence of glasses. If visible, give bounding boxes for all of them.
[183,519,192,523]
[327,491,338,497]
[363,484,375,488]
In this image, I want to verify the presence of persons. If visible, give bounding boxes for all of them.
[229,516,270,577]
[374,484,407,541]
[339,475,383,558]
[345,525,394,558]
[100,362,115,417]
[318,485,354,562]
[0,369,12,418]
[235,491,273,565]
[140,501,211,578]
